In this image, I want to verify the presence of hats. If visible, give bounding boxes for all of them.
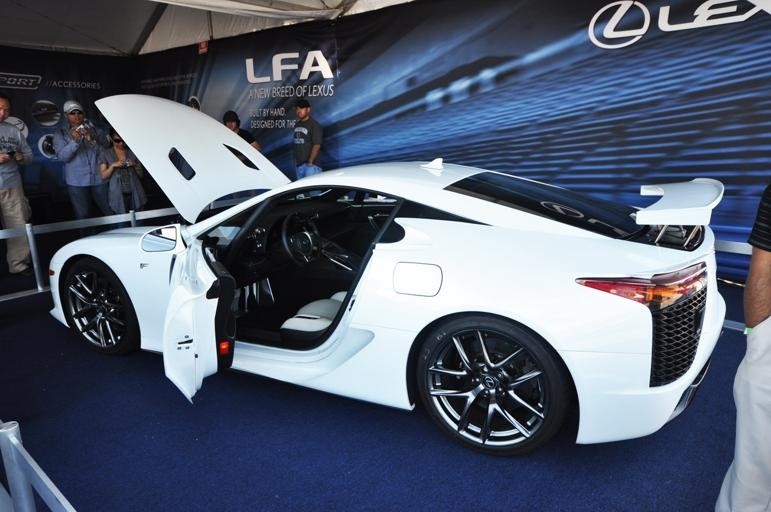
[64,100,84,114]
[294,99,311,109]
[223,111,239,123]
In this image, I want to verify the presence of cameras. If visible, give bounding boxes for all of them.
[123,159,131,168]
[76,118,94,137]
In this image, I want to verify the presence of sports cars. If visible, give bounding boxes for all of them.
[45,92,727,461]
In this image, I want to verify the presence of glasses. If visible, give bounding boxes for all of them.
[113,138,124,144]
[69,111,81,116]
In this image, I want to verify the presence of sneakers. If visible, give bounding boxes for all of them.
[9,263,29,273]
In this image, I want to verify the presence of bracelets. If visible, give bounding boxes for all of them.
[308,160,314,164]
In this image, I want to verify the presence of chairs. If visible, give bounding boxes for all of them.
[279,290,351,336]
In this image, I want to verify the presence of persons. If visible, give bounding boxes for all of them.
[53,100,113,235]
[714,183,771,512]
[223,110,262,198]
[291,98,325,196]
[97,128,149,227]
[1,94,36,278]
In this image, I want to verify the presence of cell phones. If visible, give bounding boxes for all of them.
[7,150,16,160]
